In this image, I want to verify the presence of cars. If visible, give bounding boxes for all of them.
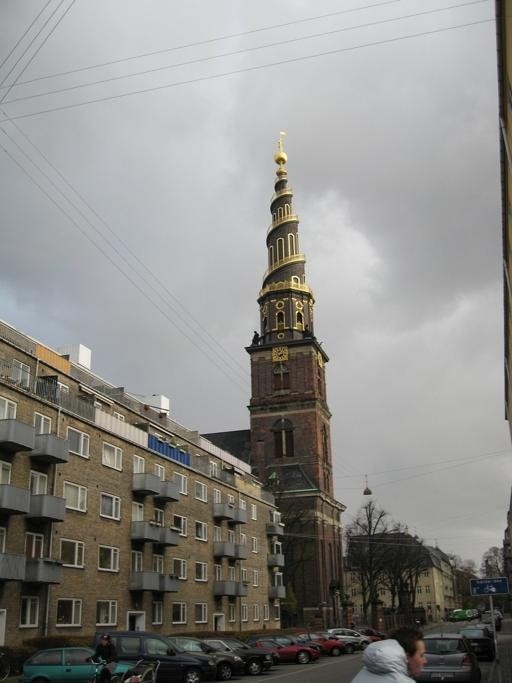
[417,630,483,682]
[91,624,390,682]
[447,605,505,660]
[19,644,159,681]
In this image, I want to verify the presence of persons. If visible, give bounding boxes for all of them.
[343,625,428,682]
[85,631,121,682]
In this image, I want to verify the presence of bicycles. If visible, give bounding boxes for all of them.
[0,652,11,681]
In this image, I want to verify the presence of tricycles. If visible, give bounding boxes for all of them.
[83,653,163,682]
